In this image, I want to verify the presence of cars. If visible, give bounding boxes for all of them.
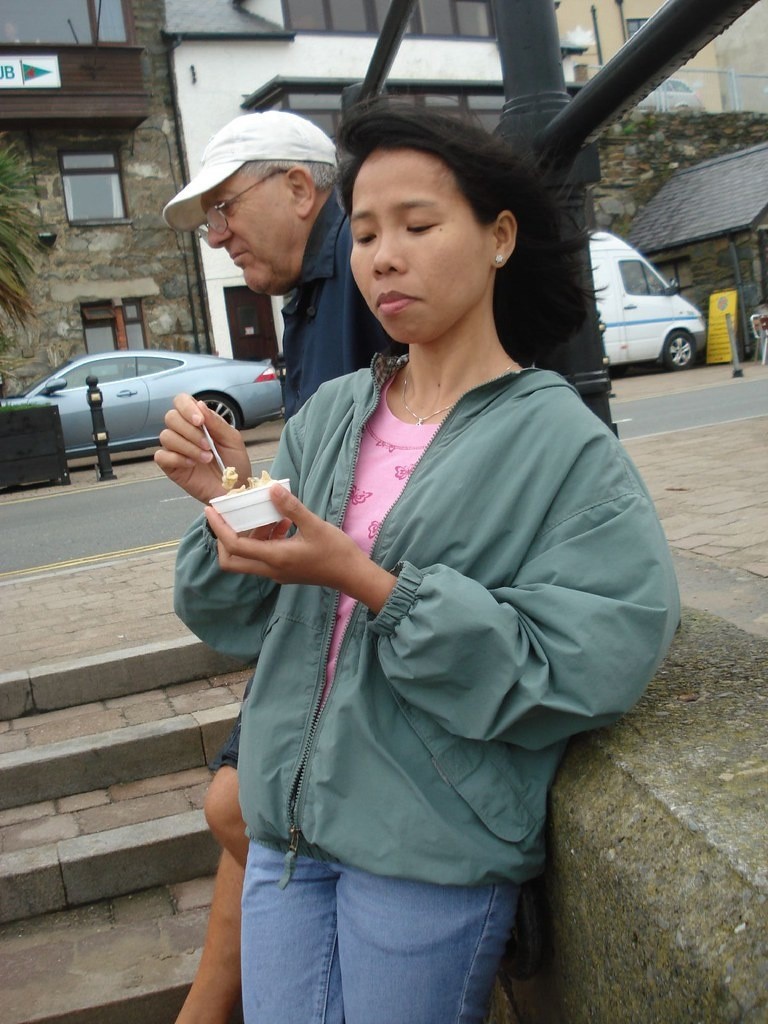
[0,349,287,456]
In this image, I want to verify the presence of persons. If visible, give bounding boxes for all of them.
[154,102,681,1024]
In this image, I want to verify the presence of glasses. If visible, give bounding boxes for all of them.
[188,167,286,239]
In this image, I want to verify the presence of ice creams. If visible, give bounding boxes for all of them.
[209,467,292,533]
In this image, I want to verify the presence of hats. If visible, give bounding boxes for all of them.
[162,111,338,231]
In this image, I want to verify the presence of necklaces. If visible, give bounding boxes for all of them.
[402,363,518,425]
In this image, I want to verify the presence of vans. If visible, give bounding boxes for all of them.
[588,229,707,376]
